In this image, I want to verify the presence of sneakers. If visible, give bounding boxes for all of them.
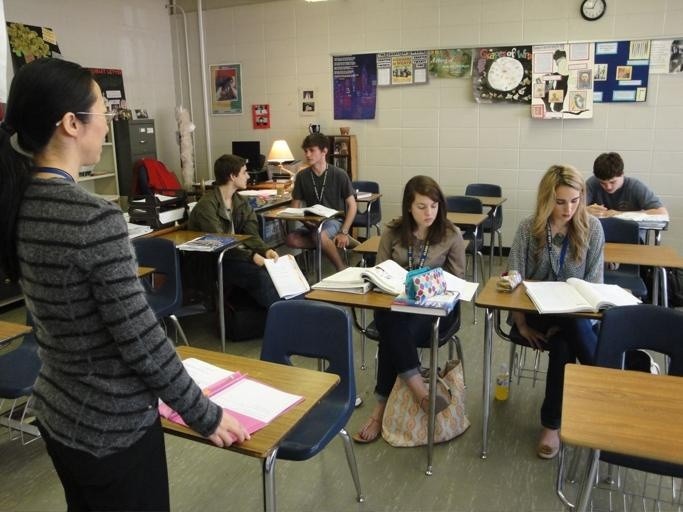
[346,234,361,249]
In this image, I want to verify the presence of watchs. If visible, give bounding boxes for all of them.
[337,229,349,235]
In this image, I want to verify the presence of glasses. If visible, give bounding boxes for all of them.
[56,109,115,127]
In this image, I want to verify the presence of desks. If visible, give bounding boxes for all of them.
[596,207,668,302]
[160,230,253,354]
[558,362,682,510]
[262,205,346,282]
[355,189,382,241]
[353,234,472,254]
[305,276,456,478]
[466,194,510,279]
[602,240,681,308]
[472,277,634,459]
[0,319,33,348]
[156,344,343,511]
[445,211,491,325]
[137,266,157,288]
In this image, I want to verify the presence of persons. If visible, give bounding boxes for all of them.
[583,152,669,280]
[284,134,362,269]
[578,72,589,89]
[186,154,308,314]
[502,164,606,459]
[352,177,469,443]
[0,57,253,510]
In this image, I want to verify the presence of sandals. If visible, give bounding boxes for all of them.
[538,427,559,458]
[420,394,449,416]
[352,416,382,443]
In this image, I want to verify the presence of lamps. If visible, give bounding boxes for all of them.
[267,140,295,179]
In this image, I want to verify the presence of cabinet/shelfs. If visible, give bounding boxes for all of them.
[76,112,120,200]
[298,135,358,180]
[111,119,157,197]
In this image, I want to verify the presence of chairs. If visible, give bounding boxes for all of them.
[598,217,647,301]
[507,323,602,390]
[466,183,504,264]
[257,213,268,242]
[1,305,45,447]
[362,253,468,396]
[444,195,487,288]
[350,182,381,241]
[129,236,191,346]
[260,300,366,505]
[585,305,681,492]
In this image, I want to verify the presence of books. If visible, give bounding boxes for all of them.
[175,234,236,252]
[321,259,413,296]
[355,191,372,200]
[522,276,644,316]
[279,204,338,220]
[264,254,312,301]
[442,269,479,302]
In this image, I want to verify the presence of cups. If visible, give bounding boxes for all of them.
[339,127,350,135]
[309,125,320,134]
[275,183,284,196]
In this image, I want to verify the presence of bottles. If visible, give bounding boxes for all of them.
[495,363,509,400]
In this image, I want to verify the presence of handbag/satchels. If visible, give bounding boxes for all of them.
[382,360,471,447]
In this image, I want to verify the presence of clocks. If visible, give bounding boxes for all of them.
[580,1,606,21]
